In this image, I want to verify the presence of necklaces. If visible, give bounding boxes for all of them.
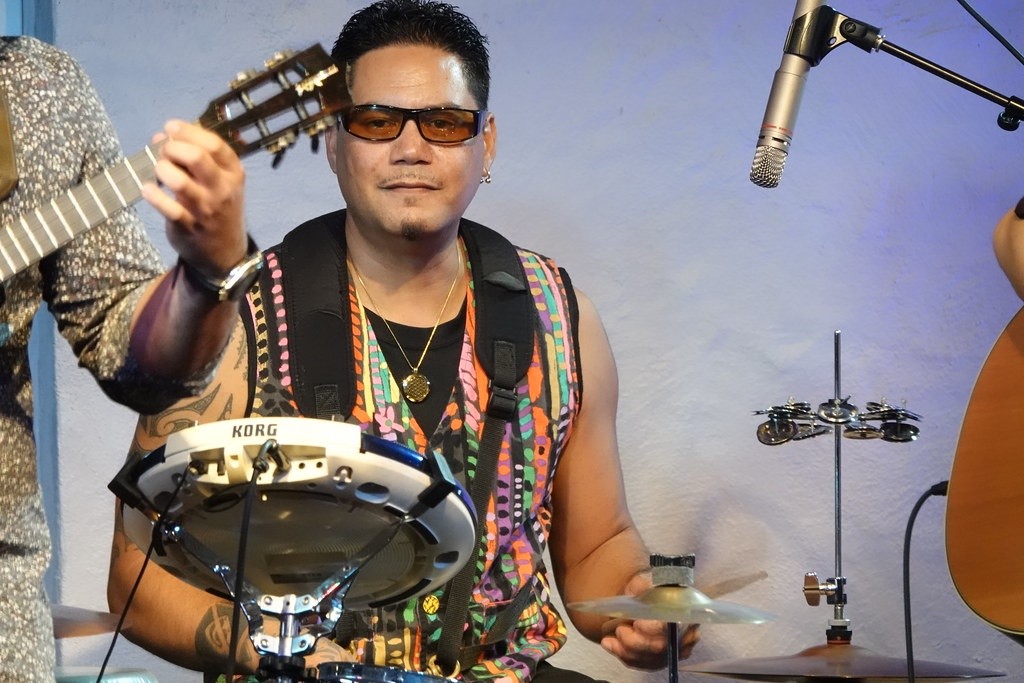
[346,239,462,404]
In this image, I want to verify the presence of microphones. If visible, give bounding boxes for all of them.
[750,0,827,187]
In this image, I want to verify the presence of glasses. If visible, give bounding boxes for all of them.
[338,104,484,144]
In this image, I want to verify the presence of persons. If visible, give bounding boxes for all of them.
[0,33,248,683]
[108,0,700,683]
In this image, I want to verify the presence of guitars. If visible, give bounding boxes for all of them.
[1,41,354,283]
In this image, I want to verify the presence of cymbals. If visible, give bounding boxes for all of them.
[566,583,768,625]
[678,645,1007,683]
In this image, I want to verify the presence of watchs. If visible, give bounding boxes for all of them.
[182,233,266,301]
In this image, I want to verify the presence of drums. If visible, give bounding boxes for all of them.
[122,415,477,614]
